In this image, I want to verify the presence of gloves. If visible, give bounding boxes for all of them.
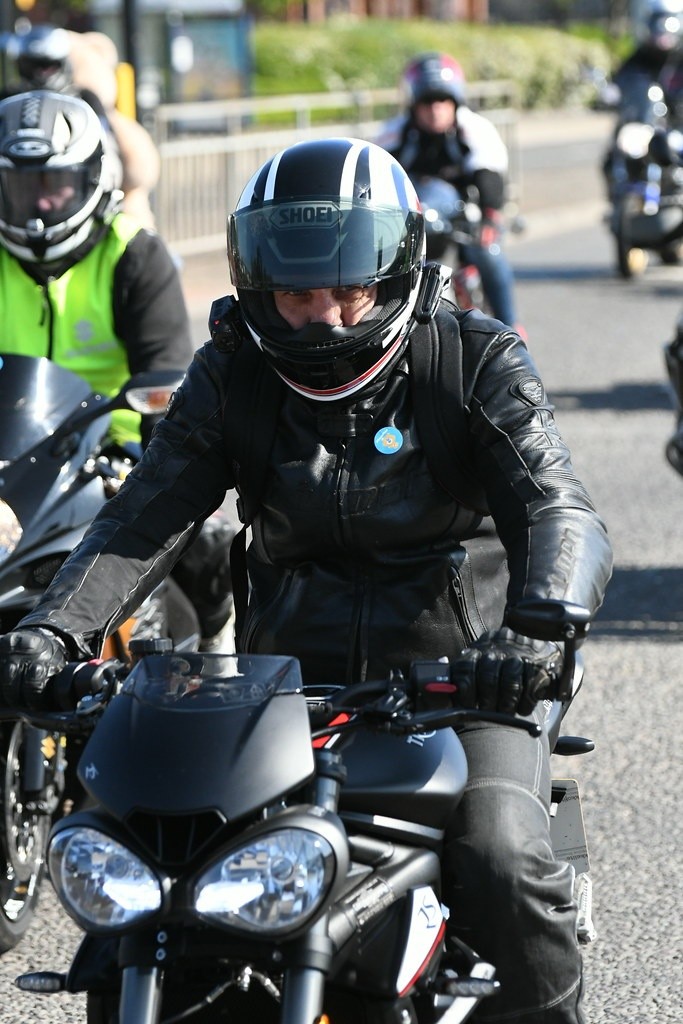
[0,625,79,712]
[449,623,568,724]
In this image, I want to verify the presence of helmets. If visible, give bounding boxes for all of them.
[1,90,116,266]
[16,22,81,96]
[406,53,466,109]
[227,138,430,405]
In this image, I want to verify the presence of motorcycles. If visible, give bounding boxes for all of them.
[416,176,485,312]
[599,88,682,276]
[2,349,234,946]
[6,659,598,1023]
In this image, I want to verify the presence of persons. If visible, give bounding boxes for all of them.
[365,50,536,344]
[2,136,614,1024]
[4,25,158,242]
[2,89,242,682]
[589,4,681,175]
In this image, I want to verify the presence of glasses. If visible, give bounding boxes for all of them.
[421,94,450,104]
